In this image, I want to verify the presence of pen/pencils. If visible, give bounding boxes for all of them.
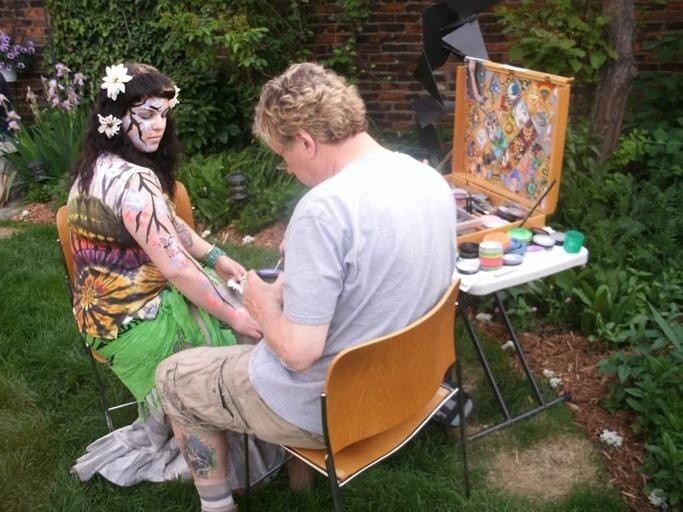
[273,255,284,274]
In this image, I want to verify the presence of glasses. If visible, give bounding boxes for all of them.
[274,141,289,172]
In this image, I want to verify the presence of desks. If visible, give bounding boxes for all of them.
[451,231,589,445]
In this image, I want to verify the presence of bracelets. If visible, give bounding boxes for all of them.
[205,245,225,269]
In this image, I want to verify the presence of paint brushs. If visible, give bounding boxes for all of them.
[470,201,489,215]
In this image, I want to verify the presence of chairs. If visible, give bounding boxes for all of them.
[56,180,196,433]
[245,278,470,511]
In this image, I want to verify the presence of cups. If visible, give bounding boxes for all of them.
[563,231,584,252]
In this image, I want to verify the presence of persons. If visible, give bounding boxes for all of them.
[154,60,459,510]
[67,55,286,487]
